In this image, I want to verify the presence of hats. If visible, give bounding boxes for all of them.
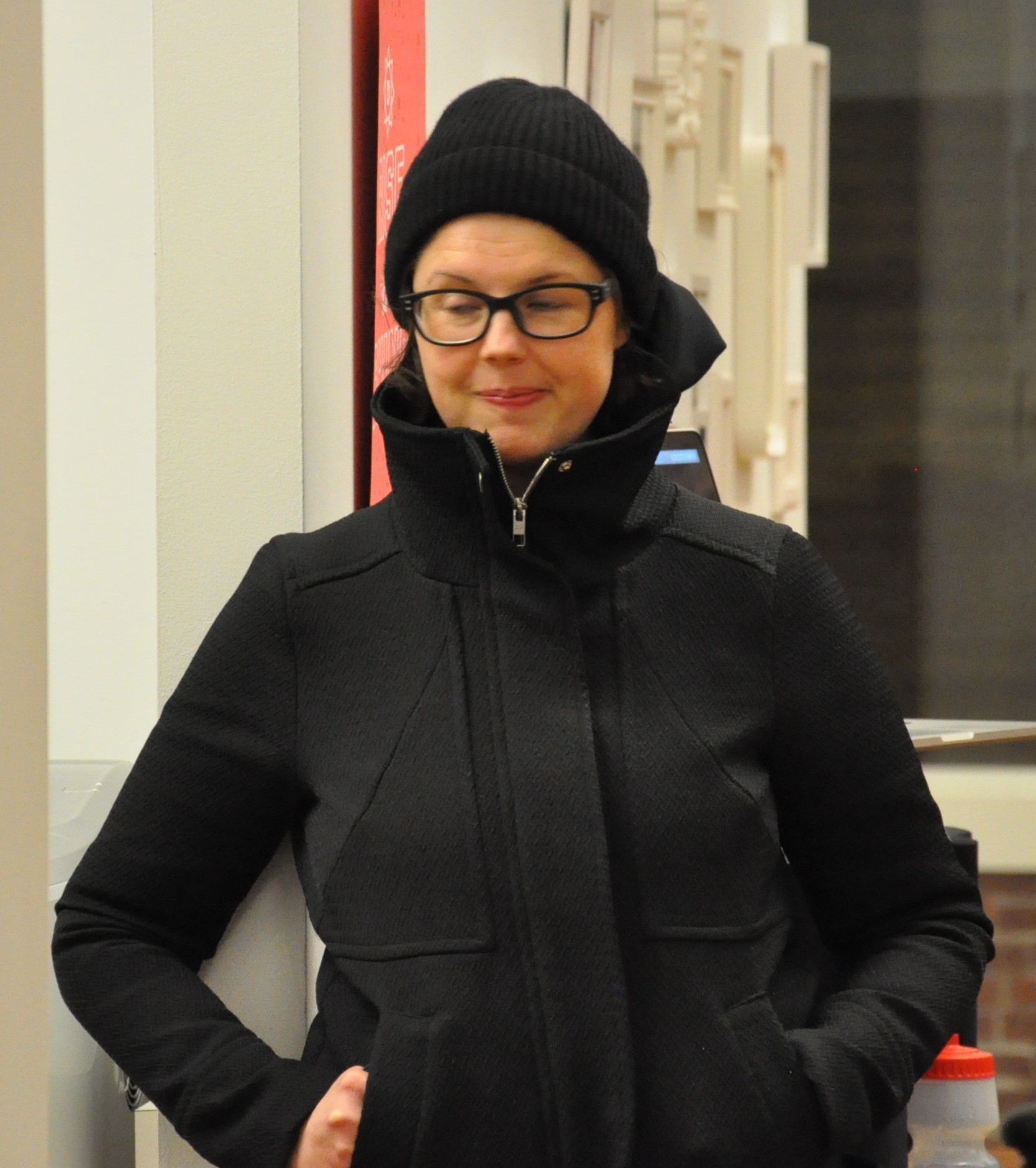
[385,77,652,333]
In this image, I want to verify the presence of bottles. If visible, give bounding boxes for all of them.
[906,1033,1000,1168]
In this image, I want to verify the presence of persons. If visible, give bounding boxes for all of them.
[53,79,995,1168]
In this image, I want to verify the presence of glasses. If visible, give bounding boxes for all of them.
[398,279,616,348]
